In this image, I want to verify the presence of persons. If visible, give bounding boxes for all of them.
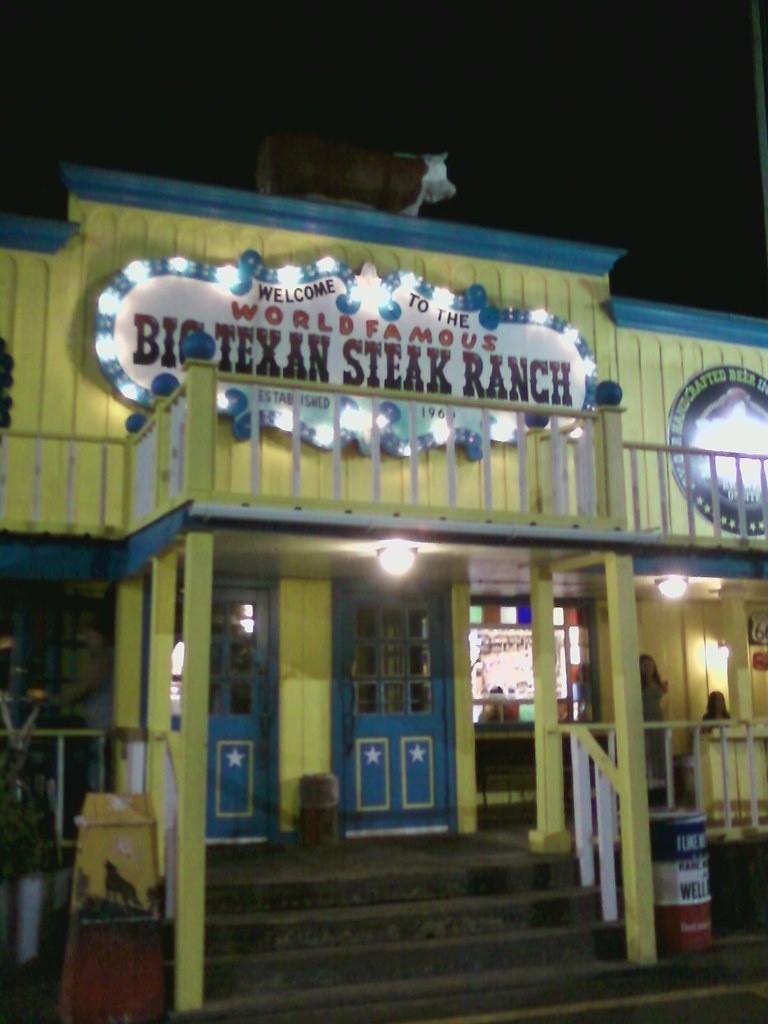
[478,687,517,720]
[640,655,668,734]
[53,618,113,736]
[702,691,729,733]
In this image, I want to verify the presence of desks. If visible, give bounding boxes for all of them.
[700,727,768,836]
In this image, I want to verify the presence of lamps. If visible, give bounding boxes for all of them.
[376,547,418,574]
[656,576,689,599]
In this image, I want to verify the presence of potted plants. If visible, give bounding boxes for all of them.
[0,749,71,967]
